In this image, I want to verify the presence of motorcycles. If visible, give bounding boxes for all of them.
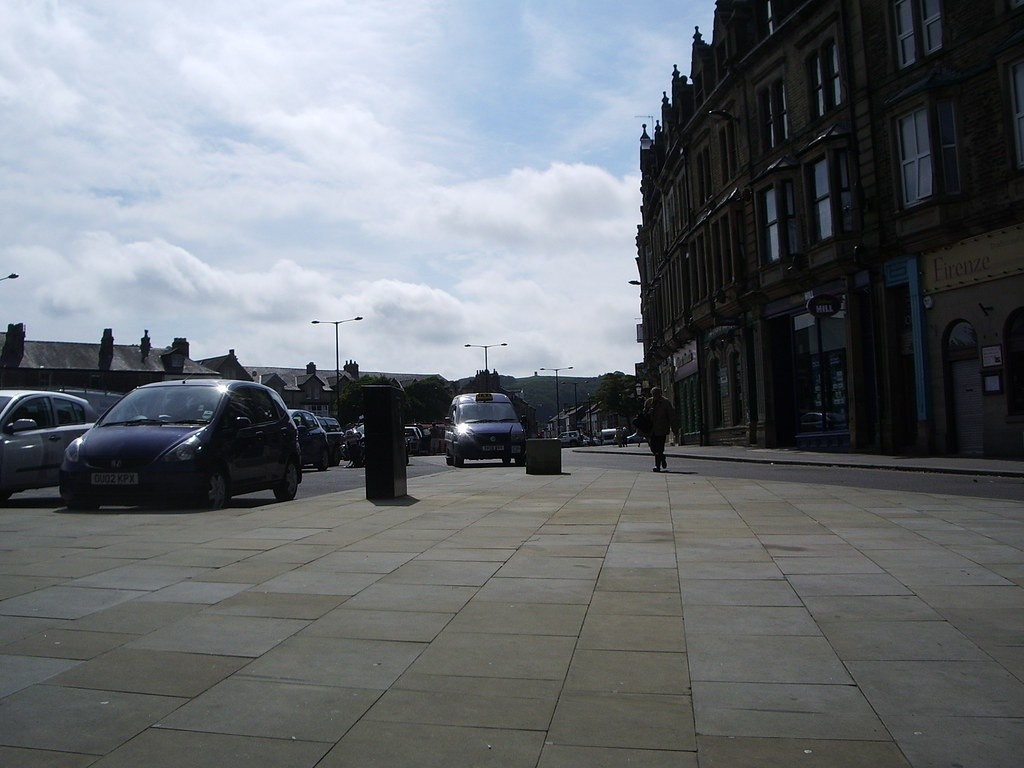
[343,414,365,468]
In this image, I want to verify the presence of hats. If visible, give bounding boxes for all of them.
[623,427,626,429]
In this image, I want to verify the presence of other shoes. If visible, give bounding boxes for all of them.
[662,459,667,468]
[653,467,660,472]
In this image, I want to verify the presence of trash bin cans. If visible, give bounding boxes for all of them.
[360,377,408,499]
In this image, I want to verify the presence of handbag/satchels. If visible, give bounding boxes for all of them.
[632,410,653,434]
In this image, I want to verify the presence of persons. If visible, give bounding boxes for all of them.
[643,386,679,472]
[616,426,628,447]
[428,421,440,455]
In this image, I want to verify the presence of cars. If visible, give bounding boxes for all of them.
[57,380,303,513]
[51,389,140,416]
[557,428,649,448]
[285,408,330,471]
[315,416,344,467]
[356,423,444,457]
[0,389,101,505]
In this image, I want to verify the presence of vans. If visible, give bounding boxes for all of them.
[444,391,528,469]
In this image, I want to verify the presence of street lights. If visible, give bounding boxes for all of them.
[540,366,574,436]
[561,380,589,431]
[464,342,508,393]
[310,316,364,418]
